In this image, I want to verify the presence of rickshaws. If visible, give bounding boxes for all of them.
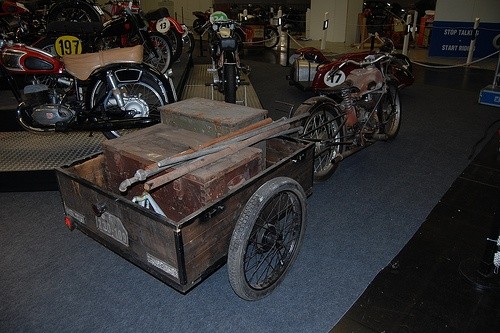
[54,102,350,303]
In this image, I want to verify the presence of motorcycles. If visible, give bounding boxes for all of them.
[0,1,280,130]
[285,27,417,181]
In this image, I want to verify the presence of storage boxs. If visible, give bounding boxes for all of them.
[100,122,264,225]
[157,97,269,140]
